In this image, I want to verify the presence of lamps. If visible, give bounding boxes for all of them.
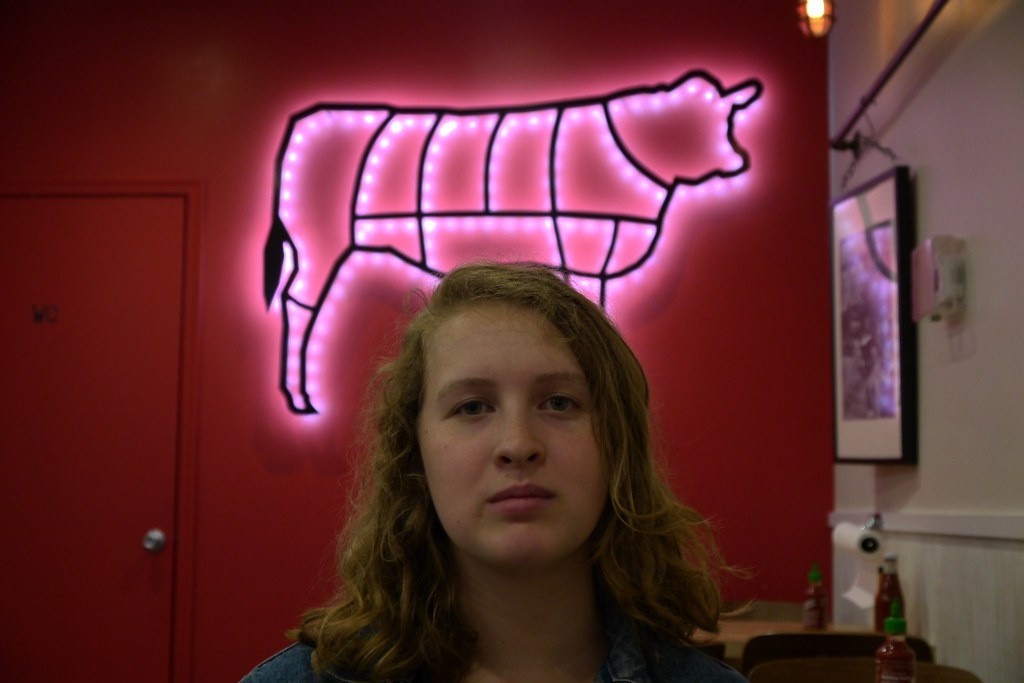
[794,0,836,40]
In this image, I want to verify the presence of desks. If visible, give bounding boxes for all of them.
[690,620,875,659]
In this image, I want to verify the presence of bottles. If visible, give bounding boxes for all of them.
[874,599,915,683]
[874,554,905,633]
[803,563,828,631]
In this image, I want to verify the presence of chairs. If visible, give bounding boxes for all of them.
[743,633,980,683]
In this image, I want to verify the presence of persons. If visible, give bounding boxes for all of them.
[244,261,756,682]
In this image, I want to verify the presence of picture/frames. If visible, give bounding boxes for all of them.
[830,165,918,466]
